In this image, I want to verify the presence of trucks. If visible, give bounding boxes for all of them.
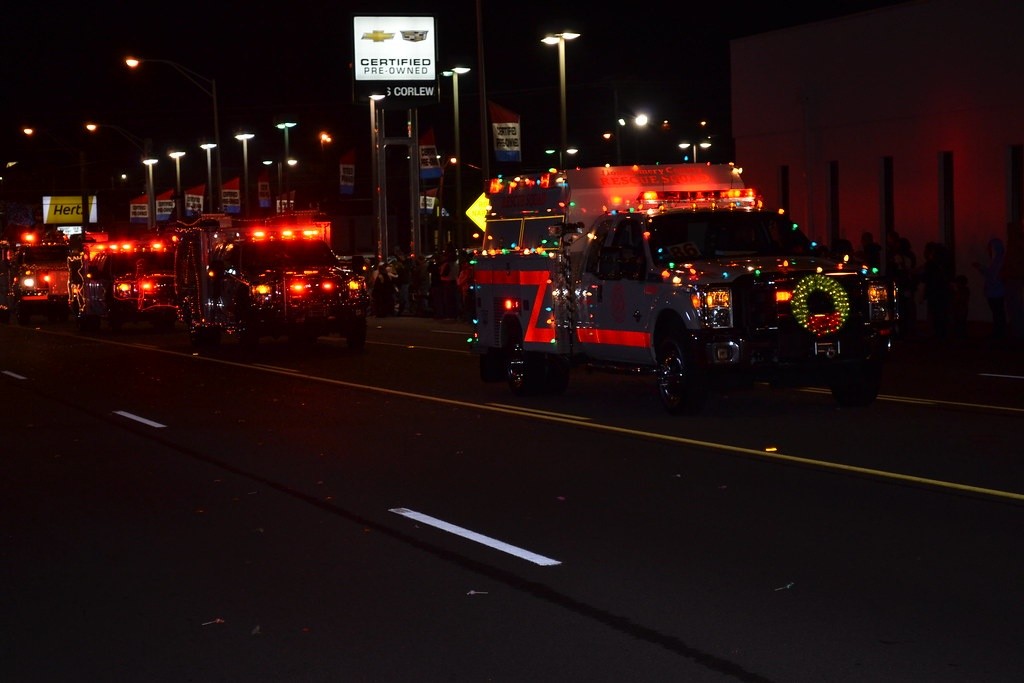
[470,163,893,419]
[170,208,372,360]
[0,225,187,330]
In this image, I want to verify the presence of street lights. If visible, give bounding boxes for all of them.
[168,149,187,219]
[142,156,160,229]
[540,27,581,152]
[86,124,153,231]
[678,141,712,163]
[21,125,87,235]
[367,80,387,261]
[235,126,256,217]
[124,55,225,214]
[199,140,219,214]
[275,116,298,211]
[545,148,578,170]
[441,66,472,246]
